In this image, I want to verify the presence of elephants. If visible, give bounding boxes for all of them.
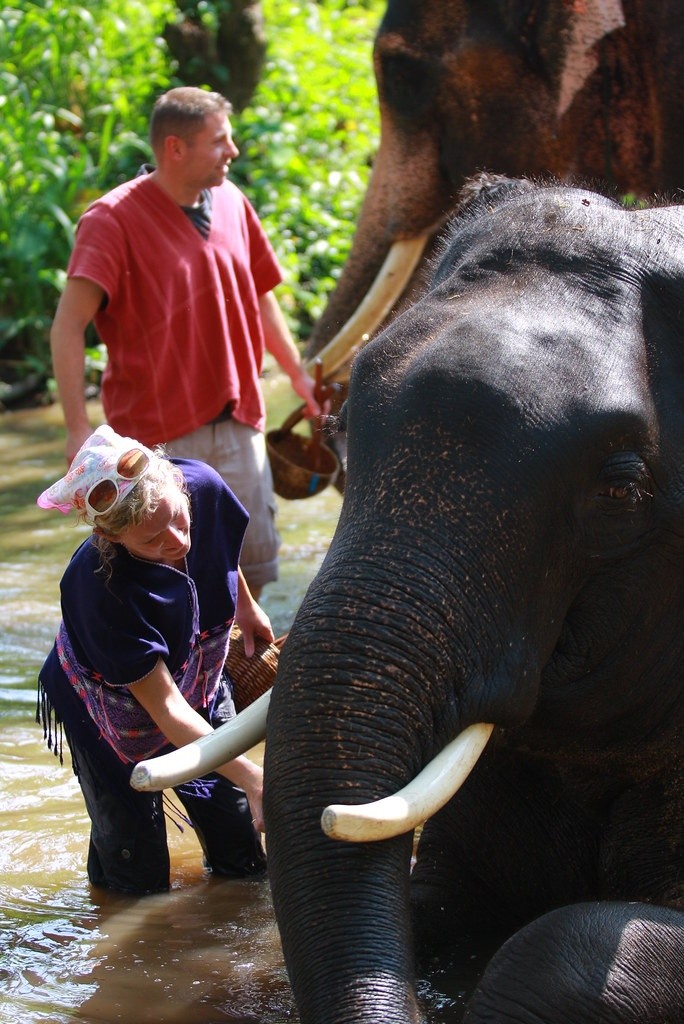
[299,1,682,388]
[128,170,684,1024]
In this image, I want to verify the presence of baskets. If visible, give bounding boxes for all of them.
[224,625,288,710]
[264,360,340,500]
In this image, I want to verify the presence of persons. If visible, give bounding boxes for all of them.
[49,86,332,604]
[35,425,278,898]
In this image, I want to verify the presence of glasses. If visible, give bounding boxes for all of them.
[85,448,150,521]
